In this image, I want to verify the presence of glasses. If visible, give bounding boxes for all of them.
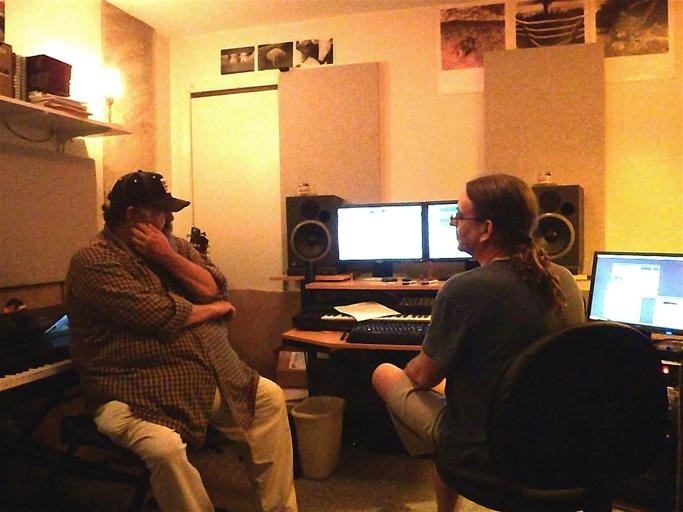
[448,214,485,226]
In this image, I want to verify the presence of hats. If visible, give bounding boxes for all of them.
[108,169,190,212]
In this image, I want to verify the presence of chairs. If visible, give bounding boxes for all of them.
[37,415,155,511]
[436,320,669,511]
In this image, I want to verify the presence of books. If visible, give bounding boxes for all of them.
[28,89,91,120]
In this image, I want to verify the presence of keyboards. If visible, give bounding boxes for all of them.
[346,321,430,347]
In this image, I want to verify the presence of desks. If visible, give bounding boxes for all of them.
[268,271,452,397]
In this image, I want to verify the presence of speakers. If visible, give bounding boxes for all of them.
[533,183,586,276]
[284,194,345,278]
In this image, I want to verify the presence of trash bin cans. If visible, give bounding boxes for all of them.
[290,395,345,481]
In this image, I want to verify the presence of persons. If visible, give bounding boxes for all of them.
[64,168,305,511]
[367,172,590,512]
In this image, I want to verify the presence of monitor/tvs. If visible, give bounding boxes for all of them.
[335,200,427,279]
[586,248,683,337]
[425,199,481,271]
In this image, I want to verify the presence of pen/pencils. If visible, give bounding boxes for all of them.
[402,279,411,281]
[381,277,398,282]
[341,328,349,341]
[420,279,439,286]
[402,281,417,285]
